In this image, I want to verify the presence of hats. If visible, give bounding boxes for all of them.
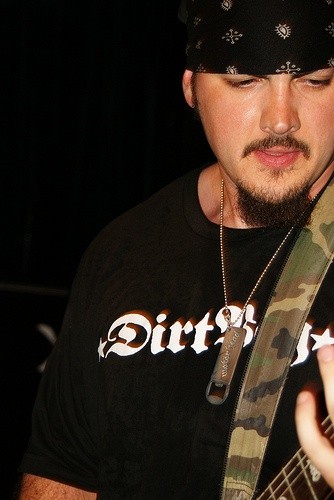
[185,0,333,73]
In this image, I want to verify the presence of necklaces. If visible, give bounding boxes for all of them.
[206,178,319,407]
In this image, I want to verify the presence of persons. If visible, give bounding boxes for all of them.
[17,0,334,500]
